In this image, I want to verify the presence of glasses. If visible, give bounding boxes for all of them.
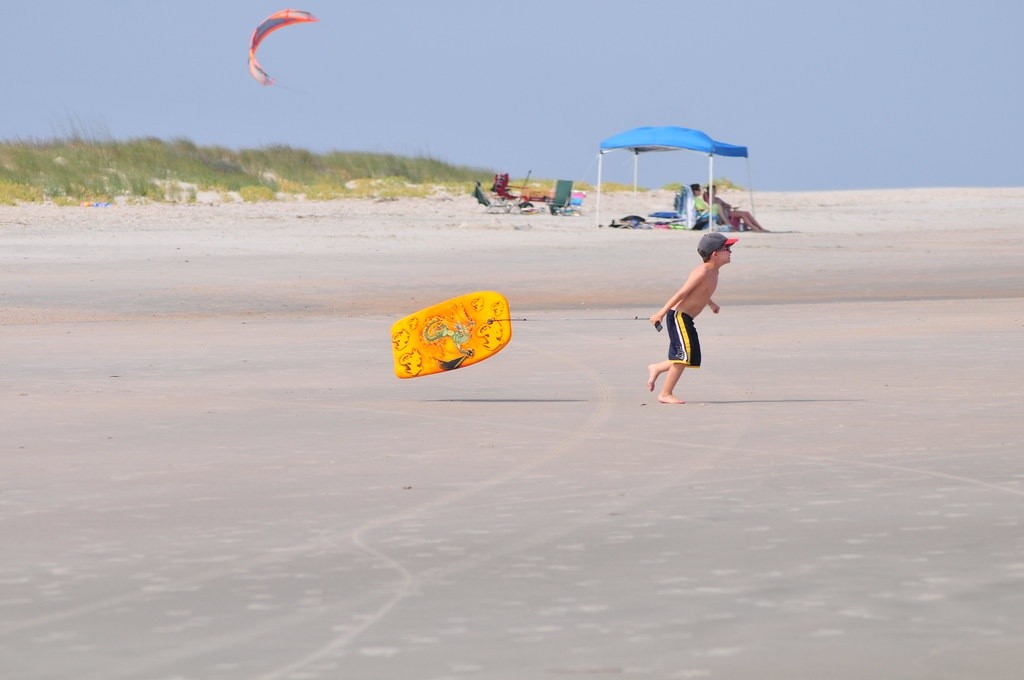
[719,246,730,251]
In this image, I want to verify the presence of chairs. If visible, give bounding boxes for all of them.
[554,190,587,217]
[543,178,574,214]
[472,179,515,214]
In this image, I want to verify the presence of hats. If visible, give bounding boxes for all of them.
[698,232,739,256]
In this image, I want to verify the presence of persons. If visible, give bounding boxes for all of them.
[647,234,732,404]
[690,183,733,228]
[705,184,769,231]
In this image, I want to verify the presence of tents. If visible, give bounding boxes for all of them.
[595,125,756,234]
